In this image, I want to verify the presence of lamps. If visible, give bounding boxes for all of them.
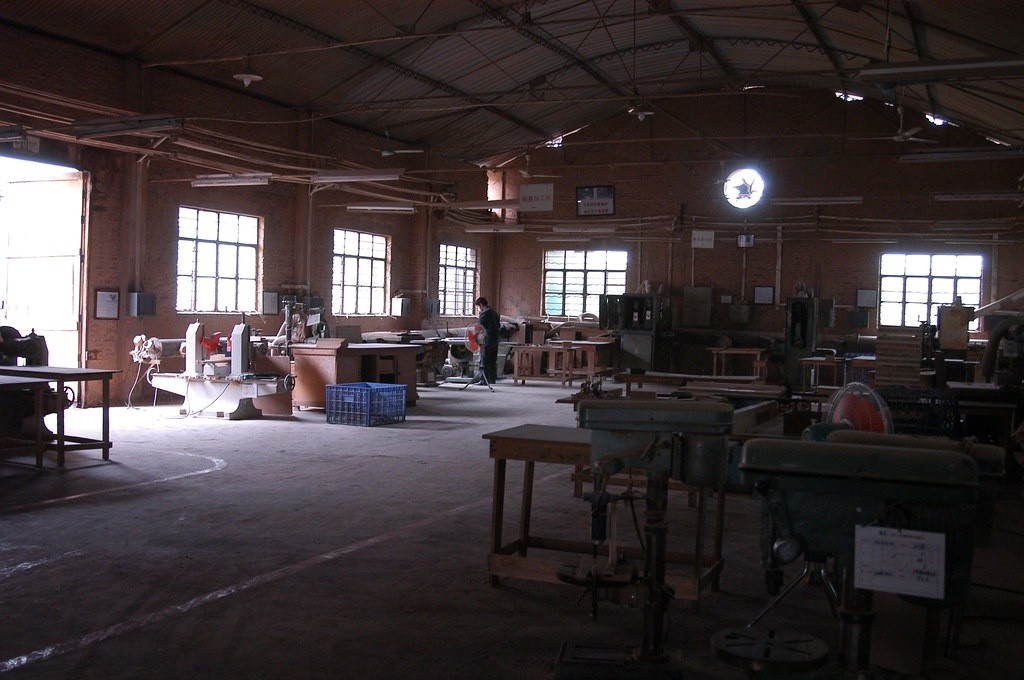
[935,192,1024,202]
[770,197,863,205]
[346,202,416,213]
[552,224,615,234]
[898,146,1024,162]
[234,59,263,87]
[191,173,274,187]
[463,223,526,233]
[311,169,401,182]
[629,98,656,122]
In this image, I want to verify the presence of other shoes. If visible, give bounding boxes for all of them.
[474,380,495,385]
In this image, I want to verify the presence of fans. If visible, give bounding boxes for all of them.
[848,105,939,144]
[799,381,893,442]
[516,145,561,179]
[370,131,425,156]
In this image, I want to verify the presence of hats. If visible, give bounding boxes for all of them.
[475,297,487,305]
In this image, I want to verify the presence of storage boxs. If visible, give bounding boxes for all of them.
[326,383,409,428]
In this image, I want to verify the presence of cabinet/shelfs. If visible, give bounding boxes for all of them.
[289,338,422,412]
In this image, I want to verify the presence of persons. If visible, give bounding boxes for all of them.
[474,297,501,386]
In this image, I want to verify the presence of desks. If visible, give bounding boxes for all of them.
[0,365,123,472]
[481,341,877,604]
[888,398,1017,487]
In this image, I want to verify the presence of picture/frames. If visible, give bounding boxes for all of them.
[855,289,877,308]
[94,288,120,320]
[576,184,615,216]
[754,286,774,305]
